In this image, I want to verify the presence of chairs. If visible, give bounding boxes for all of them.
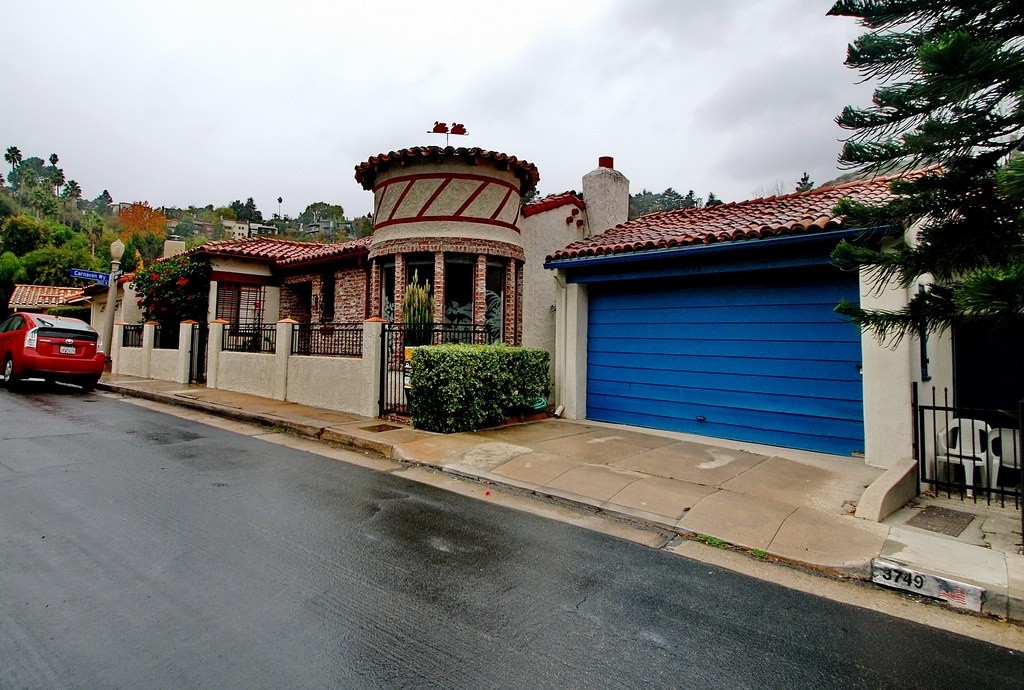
[984,426,1024,498]
[933,417,995,500]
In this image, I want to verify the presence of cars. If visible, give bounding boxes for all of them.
[0,311,107,391]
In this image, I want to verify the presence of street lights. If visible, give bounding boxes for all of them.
[103,237,127,372]
